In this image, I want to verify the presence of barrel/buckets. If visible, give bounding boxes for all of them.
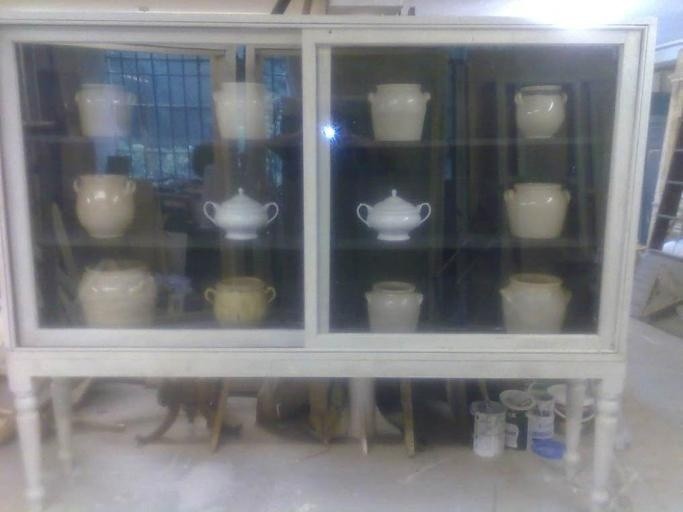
[546,384,596,435]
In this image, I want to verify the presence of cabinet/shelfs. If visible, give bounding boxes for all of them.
[0,10,657,510]
[630,44,682,338]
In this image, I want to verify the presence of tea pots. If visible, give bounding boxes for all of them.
[204,187,281,241]
[355,186,432,241]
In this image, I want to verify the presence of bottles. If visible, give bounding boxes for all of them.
[362,280,422,333]
[69,172,139,240]
[503,183,573,241]
[203,276,276,331]
[76,260,159,329]
[514,84,570,139]
[498,273,573,334]
[367,83,429,141]
[73,81,135,138]
[212,83,272,139]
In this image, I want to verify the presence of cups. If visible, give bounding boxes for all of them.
[469,384,592,456]
